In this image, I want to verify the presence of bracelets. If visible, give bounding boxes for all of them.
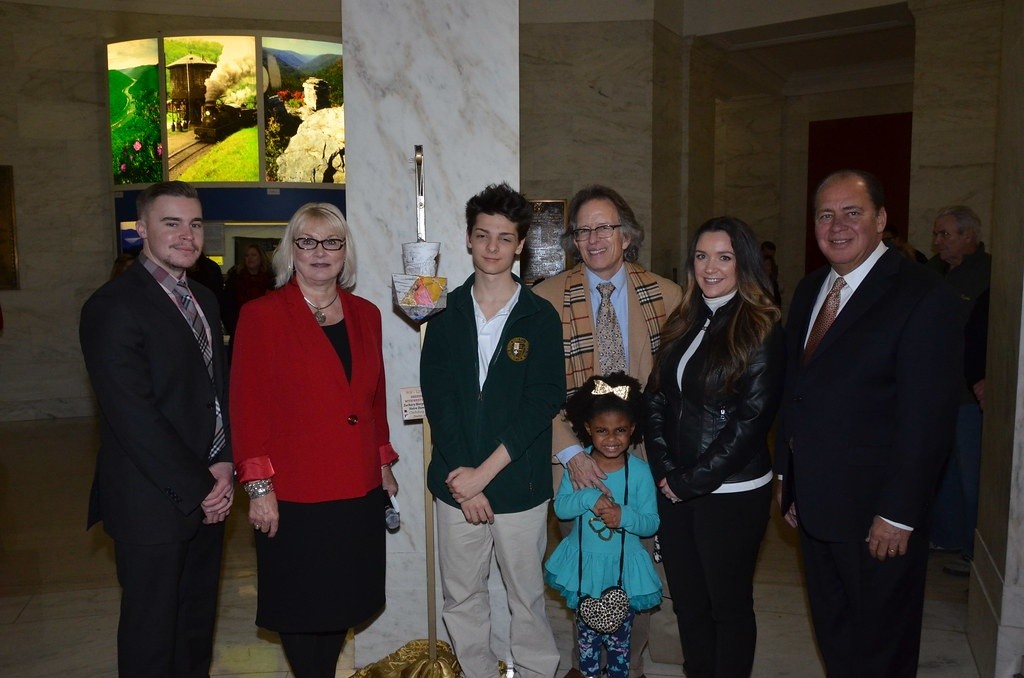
[244,478,275,500]
[382,463,392,470]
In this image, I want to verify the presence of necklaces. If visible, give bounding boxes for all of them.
[303,292,339,325]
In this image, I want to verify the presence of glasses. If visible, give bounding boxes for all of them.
[293,236,347,251]
[571,222,623,241]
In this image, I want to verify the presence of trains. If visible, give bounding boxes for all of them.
[194,99,258,143]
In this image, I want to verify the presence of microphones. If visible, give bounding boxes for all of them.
[383,489,400,529]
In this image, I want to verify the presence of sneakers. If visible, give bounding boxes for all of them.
[943,553,974,575]
[927,541,963,551]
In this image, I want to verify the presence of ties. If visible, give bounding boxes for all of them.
[804,276,846,370]
[174,279,227,461]
[596,282,628,374]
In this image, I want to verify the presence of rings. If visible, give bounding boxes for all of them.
[224,495,230,502]
[670,497,678,503]
[888,549,898,553]
[255,524,261,530]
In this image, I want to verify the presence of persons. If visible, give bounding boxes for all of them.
[775,166,945,678]
[541,369,664,678]
[183,250,223,297]
[644,213,784,678]
[882,224,929,265]
[419,183,562,678]
[758,240,781,308]
[532,184,687,678]
[226,203,399,678]
[219,242,275,363]
[111,252,137,280]
[75,180,242,677]
[920,202,990,577]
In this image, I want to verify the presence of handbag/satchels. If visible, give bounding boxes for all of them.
[577,585,626,634]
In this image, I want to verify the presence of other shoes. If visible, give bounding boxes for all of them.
[564,667,586,678]
